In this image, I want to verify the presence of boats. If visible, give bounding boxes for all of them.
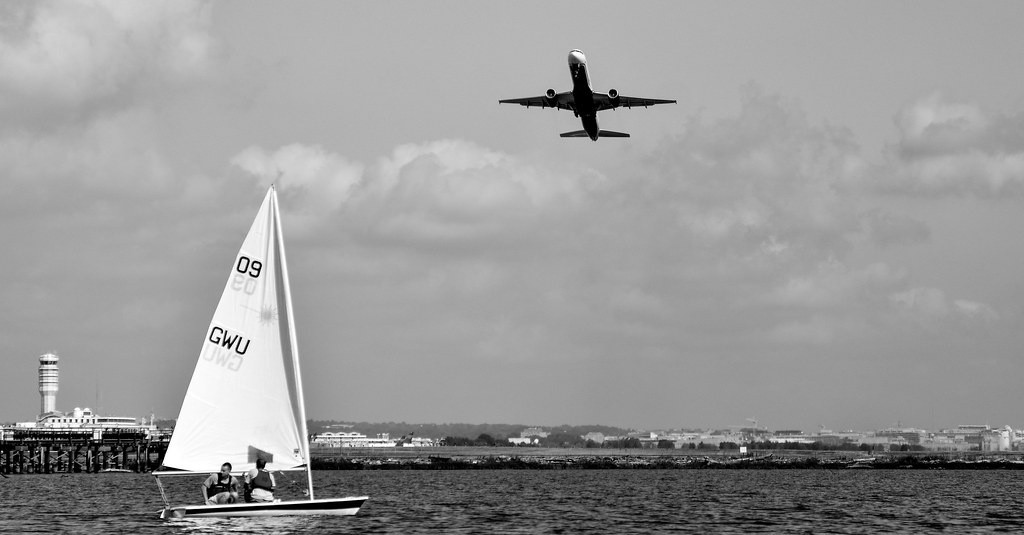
[102,468,133,473]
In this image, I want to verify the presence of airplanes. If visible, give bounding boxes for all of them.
[498,49,678,142]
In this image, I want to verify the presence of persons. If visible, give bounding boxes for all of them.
[242,459,276,503]
[201,462,240,505]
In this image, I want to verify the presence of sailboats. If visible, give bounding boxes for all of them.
[150,183,371,517]
